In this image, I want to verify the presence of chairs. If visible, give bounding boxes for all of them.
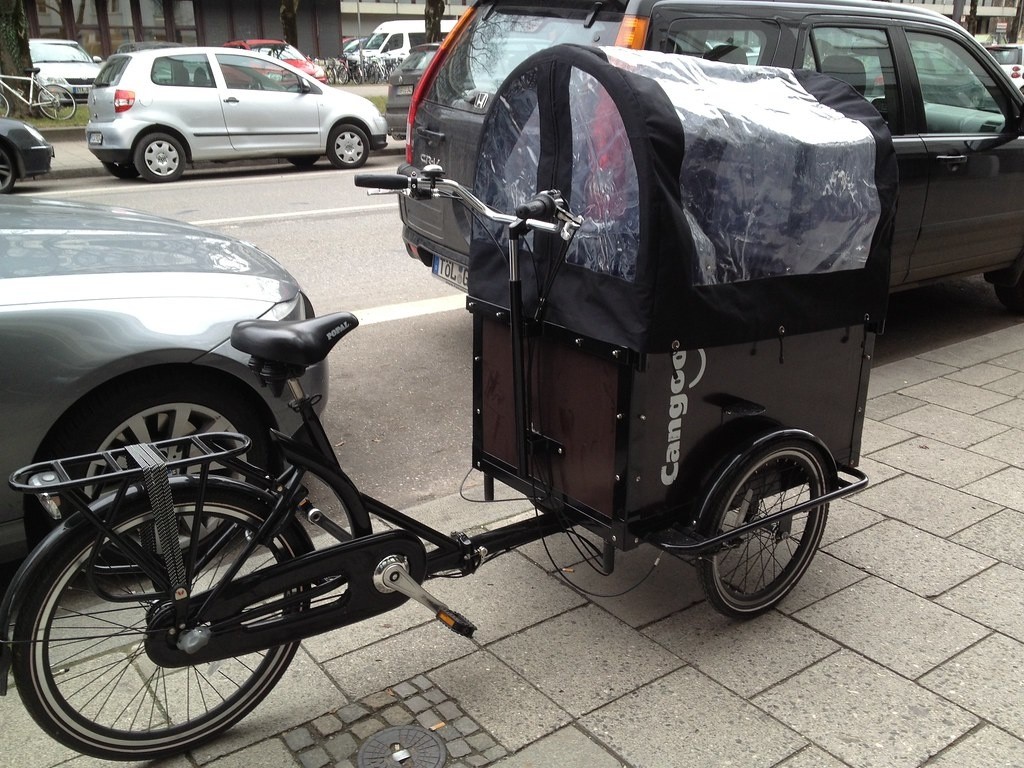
[194,68,207,87]
[822,55,866,96]
[706,45,749,66]
[175,68,189,86]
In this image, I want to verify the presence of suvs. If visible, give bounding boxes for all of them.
[399,1,1024,313]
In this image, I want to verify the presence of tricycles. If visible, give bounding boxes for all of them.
[0,43,898,761]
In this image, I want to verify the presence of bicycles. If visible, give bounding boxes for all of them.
[0,67,77,123]
[324,58,400,85]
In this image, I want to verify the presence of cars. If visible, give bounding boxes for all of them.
[1,119,57,193]
[385,38,562,140]
[845,48,984,108]
[84,45,389,184]
[982,44,1023,91]
[0,193,329,586]
[219,38,330,88]
[341,36,367,56]
[25,37,105,105]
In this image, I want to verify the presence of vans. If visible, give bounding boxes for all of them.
[356,19,461,63]
